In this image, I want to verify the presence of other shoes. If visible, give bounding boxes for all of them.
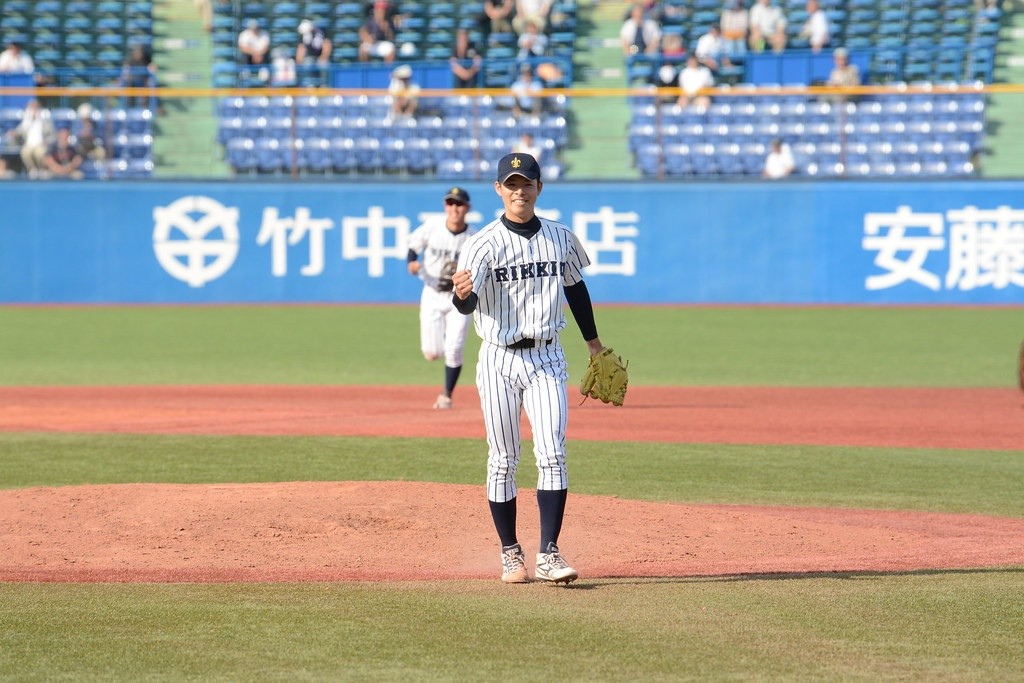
[434,395,451,408]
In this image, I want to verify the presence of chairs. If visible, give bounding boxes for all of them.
[209,0,577,177]
[628,0,1003,175]
[0,0,154,179]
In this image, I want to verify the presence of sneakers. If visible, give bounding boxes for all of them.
[535,542,578,584]
[501,543,529,583]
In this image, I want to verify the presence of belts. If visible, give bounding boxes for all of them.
[507,338,552,349]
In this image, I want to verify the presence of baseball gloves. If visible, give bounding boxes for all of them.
[579,347,629,407]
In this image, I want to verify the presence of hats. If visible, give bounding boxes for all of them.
[444,186,470,205]
[498,153,541,184]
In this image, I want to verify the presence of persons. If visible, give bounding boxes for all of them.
[408,187,479,408]
[451,154,628,585]
[389,67,421,117]
[620,0,860,179]
[449,0,558,161]
[292,20,330,88]
[0,43,155,184]
[357,0,397,64]
[234,19,272,84]
